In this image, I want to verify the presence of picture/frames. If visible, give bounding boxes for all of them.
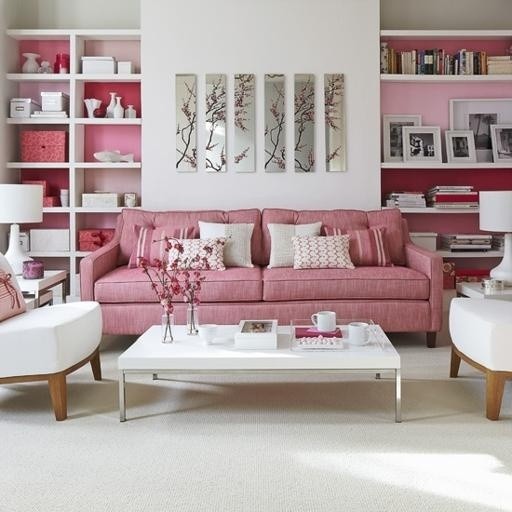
[490,120,512,163]
[448,96,512,162]
[381,112,425,162]
[398,124,444,164]
[442,128,478,163]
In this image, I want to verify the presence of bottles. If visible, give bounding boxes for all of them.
[105,91,136,118]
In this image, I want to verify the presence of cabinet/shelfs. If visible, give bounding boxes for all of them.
[380,27,512,284]
[3,28,143,303]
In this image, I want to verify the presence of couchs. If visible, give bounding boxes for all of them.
[449,290,511,425]
[78,205,445,349]
[0,248,105,422]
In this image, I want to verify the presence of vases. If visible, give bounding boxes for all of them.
[20,50,43,74]
[157,304,174,346]
[186,298,201,338]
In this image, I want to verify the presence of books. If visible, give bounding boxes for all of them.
[234,319,280,352]
[380,42,512,73]
[385,178,506,286]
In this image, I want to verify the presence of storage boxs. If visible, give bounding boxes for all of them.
[9,96,37,117]
[19,129,67,164]
[78,228,117,252]
[41,89,67,111]
[83,193,123,209]
[404,231,439,252]
[6,231,32,252]
[24,178,49,194]
[29,226,70,253]
[80,55,115,73]
[43,197,60,208]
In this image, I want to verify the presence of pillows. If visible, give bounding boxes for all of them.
[127,225,196,269]
[197,218,256,265]
[0,265,26,321]
[167,236,227,271]
[292,234,355,269]
[324,225,391,267]
[267,221,325,269]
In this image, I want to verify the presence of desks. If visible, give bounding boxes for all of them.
[13,268,68,306]
[452,274,512,303]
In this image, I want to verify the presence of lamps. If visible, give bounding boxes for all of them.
[0,178,45,274]
[474,185,512,287]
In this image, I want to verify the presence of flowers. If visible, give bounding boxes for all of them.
[172,237,225,303]
[133,247,181,312]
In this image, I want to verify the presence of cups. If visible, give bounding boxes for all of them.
[310,312,338,333]
[347,321,372,347]
